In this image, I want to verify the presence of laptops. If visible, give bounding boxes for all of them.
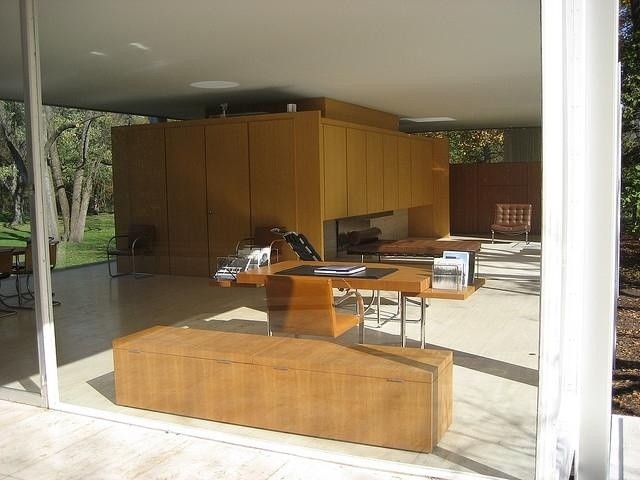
[313,266,368,276]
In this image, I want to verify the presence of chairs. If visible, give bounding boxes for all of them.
[106,224,155,279]
[0,236,61,318]
[262,274,360,344]
[490,202,533,245]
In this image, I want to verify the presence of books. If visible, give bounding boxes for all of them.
[314,265,368,273]
[430,249,476,291]
[212,246,272,280]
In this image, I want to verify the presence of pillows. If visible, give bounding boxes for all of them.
[347,227,382,247]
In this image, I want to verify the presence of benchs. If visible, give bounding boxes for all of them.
[111,324,454,453]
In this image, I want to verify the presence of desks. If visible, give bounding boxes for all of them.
[235,258,485,348]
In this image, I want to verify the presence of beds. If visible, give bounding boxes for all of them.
[347,238,481,278]
